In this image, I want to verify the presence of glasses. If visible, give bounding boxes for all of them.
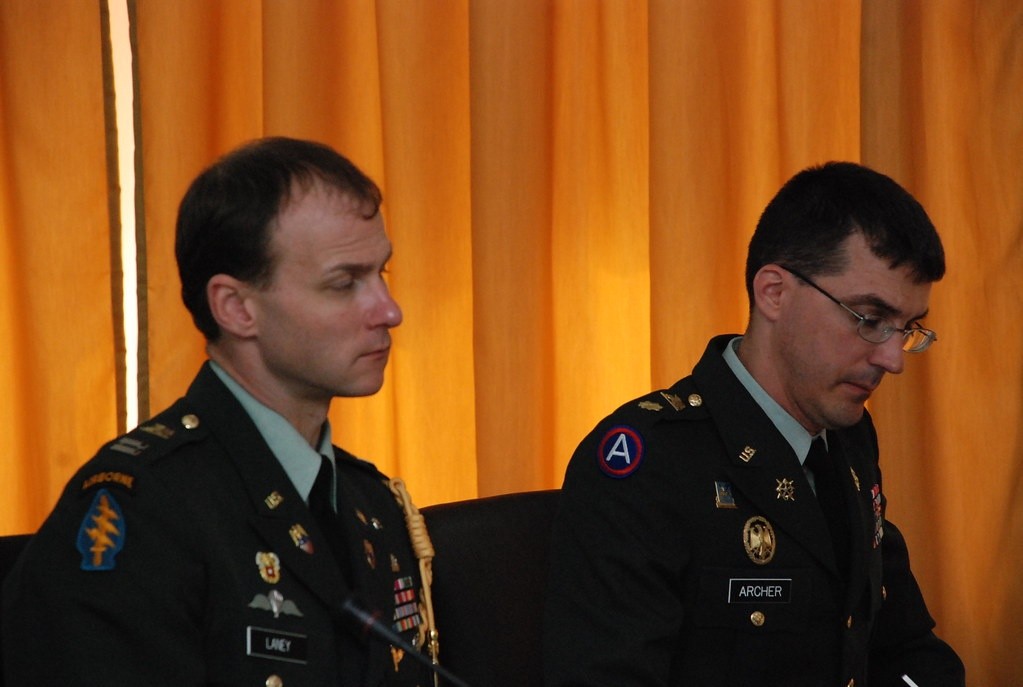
[775,264,937,354]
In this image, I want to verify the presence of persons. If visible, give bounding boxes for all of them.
[1,134,462,687]
[530,160,965,687]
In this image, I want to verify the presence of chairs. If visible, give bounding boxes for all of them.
[412,488,563,687]
